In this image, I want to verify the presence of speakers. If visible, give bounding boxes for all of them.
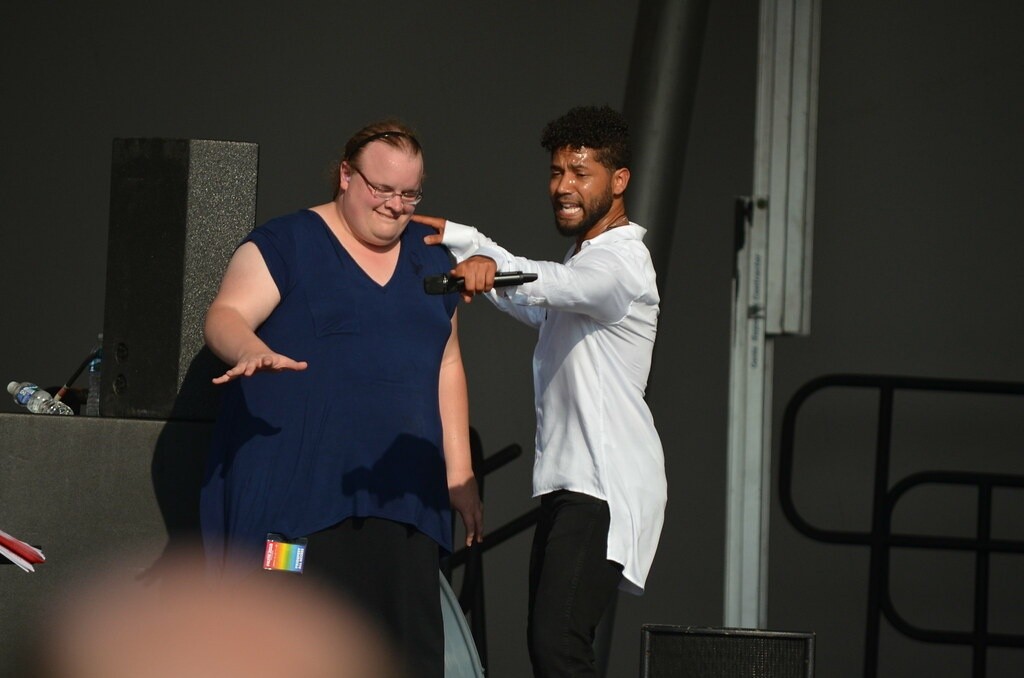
[97,137,259,421]
[639,622,817,678]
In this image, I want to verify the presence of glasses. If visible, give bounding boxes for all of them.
[347,163,423,205]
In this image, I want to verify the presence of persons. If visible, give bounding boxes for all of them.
[200,119,484,678]
[38,548,392,678]
[410,102,670,678]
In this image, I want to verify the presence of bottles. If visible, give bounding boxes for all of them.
[85,333,103,416]
[7,381,75,416]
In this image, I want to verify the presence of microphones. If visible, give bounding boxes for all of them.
[424,270,538,294]
